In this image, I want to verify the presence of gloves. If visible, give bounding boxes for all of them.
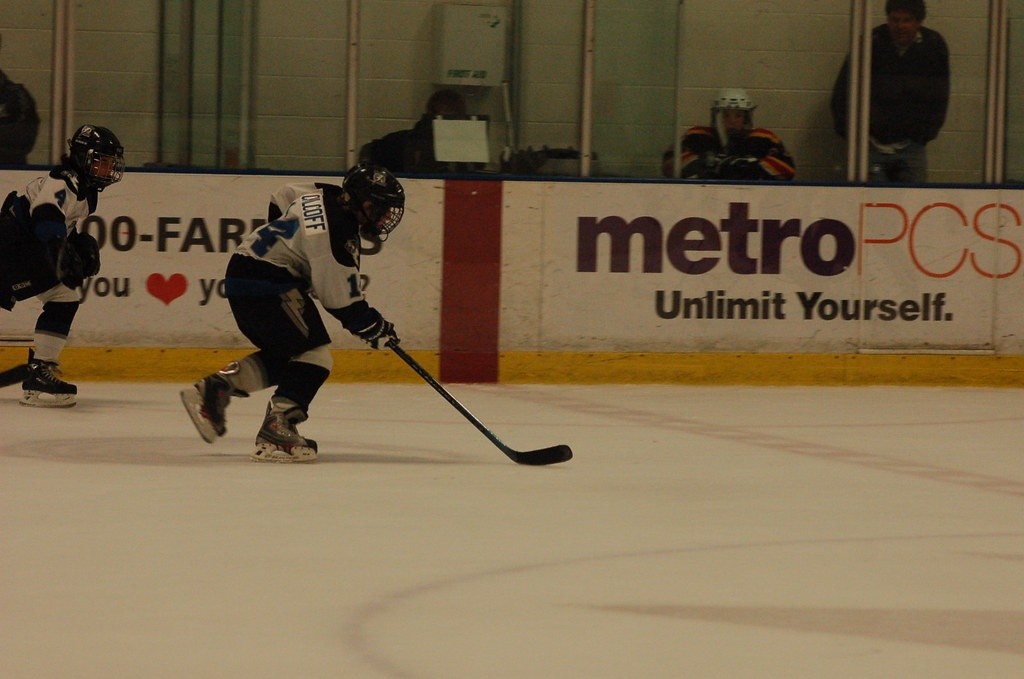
[58,231,101,289]
[358,315,400,352]
[711,154,762,181]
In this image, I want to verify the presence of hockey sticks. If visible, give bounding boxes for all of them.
[1,363,32,387]
[386,340,574,465]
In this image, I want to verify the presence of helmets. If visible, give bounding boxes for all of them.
[343,161,405,209]
[70,125,126,158]
[711,86,753,111]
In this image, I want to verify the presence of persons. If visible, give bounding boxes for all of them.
[181,163,406,466]
[0,124,125,408]
[369,90,482,174]
[663,87,798,182]
[829,0,950,183]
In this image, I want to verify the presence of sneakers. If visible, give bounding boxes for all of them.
[249,397,318,463]
[19,347,78,407]
[178,360,248,445]
